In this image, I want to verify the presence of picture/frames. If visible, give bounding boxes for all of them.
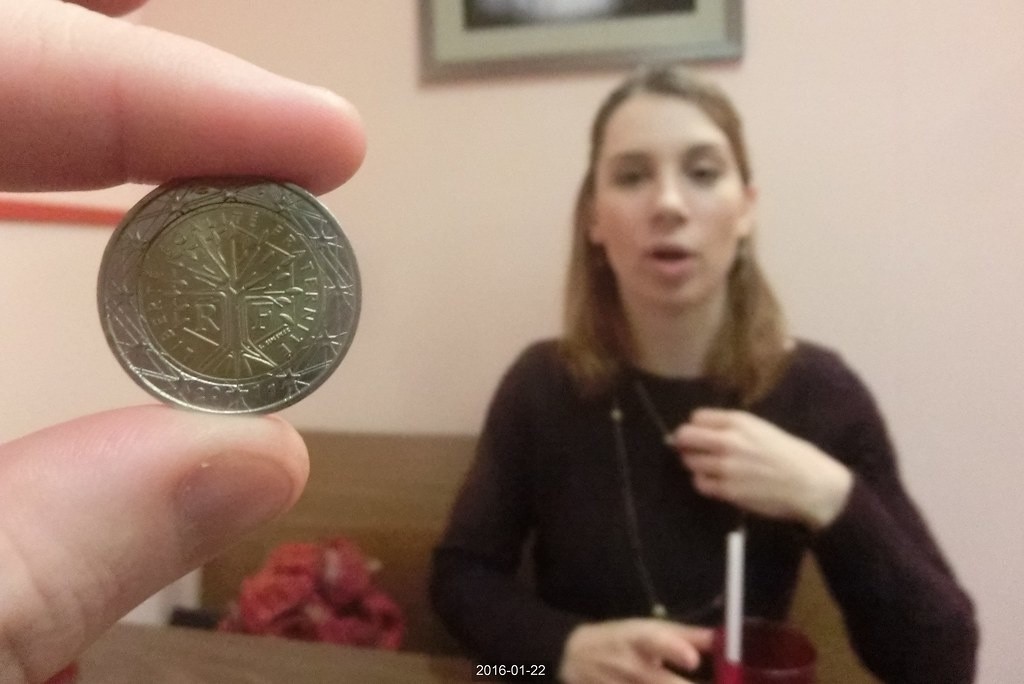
[419,0,742,84]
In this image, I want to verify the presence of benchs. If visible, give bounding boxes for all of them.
[171,433,882,684]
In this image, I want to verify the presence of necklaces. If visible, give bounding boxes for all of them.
[626,371,745,434]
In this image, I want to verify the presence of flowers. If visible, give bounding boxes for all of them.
[219,539,405,651]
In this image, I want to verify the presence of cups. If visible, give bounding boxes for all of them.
[715,622,817,684]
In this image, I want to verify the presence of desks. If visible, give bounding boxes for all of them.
[58,622,473,684]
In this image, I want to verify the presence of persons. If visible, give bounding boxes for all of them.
[424,69,983,683]
[0,1,370,680]
